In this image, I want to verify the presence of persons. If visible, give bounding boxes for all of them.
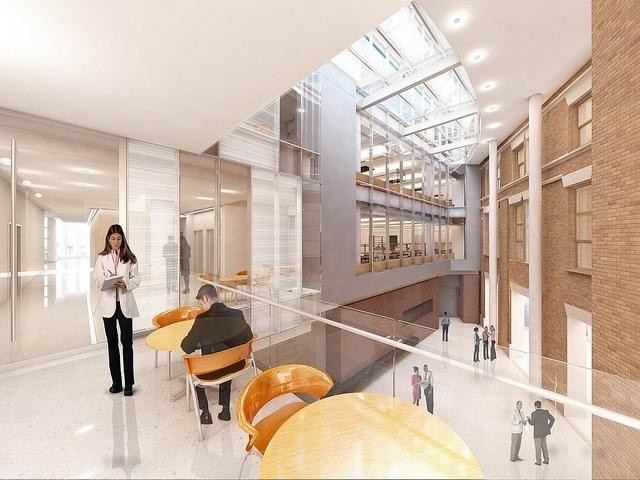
[177,236,191,294]
[411,367,422,406]
[420,364,434,414]
[473,327,482,362]
[180,284,253,424]
[93,224,141,396]
[509,401,528,462]
[482,326,490,360]
[526,401,555,466]
[490,325,497,361]
[163,235,178,295]
[440,311,450,341]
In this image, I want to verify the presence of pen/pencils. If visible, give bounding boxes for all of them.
[108,270,113,275]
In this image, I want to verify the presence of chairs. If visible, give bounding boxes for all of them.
[149,303,208,381]
[232,362,334,479]
[178,332,259,442]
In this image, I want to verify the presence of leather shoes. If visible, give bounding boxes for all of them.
[109,382,123,393]
[124,386,133,396]
[542,461,548,464]
[535,462,541,466]
[510,458,516,462]
[516,456,523,461]
[218,410,230,421]
[200,412,213,425]
